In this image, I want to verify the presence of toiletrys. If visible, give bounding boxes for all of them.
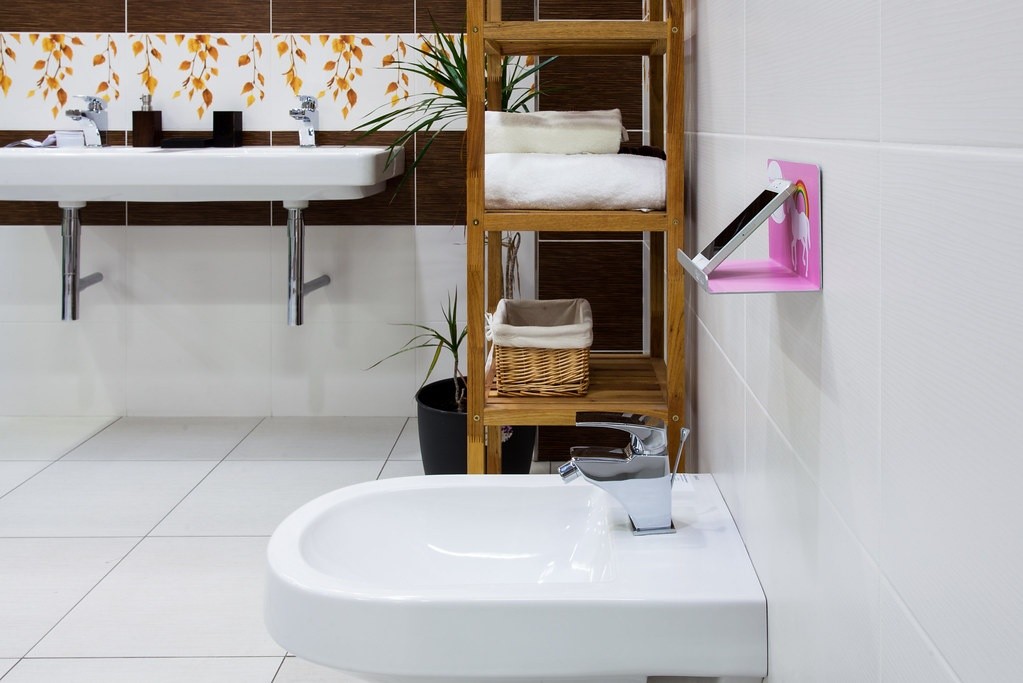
[132,93,163,147]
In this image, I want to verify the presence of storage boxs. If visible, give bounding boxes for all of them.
[490,295,592,395]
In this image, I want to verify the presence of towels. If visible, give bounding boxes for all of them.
[483,107,666,211]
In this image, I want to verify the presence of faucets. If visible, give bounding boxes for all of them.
[289,93,320,148]
[556,410,676,536]
[65,93,110,147]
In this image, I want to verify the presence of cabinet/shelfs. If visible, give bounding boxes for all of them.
[462,0,689,475]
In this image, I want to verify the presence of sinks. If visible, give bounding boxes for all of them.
[233,145,406,210]
[256,471,768,682]
[0,145,134,208]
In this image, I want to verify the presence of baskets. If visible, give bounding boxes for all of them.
[492,296,595,400]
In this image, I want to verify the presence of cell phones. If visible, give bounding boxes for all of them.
[693,178,796,273]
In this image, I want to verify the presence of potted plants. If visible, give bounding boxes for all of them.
[344,10,565,476]
